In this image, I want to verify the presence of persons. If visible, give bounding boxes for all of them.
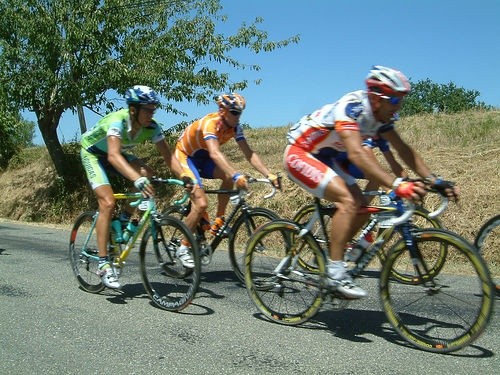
[167,91,279,268]
[281,66,461,297]
[79,84,198,290]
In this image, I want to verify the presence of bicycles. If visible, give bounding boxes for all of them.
[153,174,294,292]
[474,213,500,299]
[288,174,449,285]
[69,175,202,312]
[246,179,495,353]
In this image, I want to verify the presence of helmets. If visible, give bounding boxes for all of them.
[366,65,412,96]
[216,92,246,111]
[125,84,158,104]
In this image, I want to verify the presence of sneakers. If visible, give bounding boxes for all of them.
[321,268,369,298]
[98,261,120,289]
[175,245,196,268]
[119,211,132,222]
[210,220,232,235]
[343,243,355,262]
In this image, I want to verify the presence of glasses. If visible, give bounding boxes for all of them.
[141,107,157,114]
[380,95,403,104]
[230,111,241,116]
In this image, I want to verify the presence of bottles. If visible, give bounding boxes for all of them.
[111,214,124,244]
[208,214,225,236]
[348,232,373,261]
[122,218,139,243]
[199,211,211,231]
